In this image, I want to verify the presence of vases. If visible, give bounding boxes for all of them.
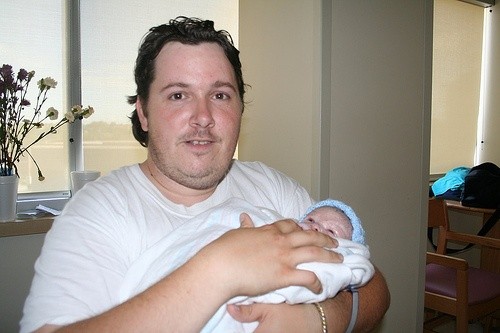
[70,169,99,195]
[0,175,18,222]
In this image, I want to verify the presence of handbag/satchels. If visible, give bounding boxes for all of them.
[432,166,472,201]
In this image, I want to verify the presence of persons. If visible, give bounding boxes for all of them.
[120,197,366,333]
[18,15,392,333]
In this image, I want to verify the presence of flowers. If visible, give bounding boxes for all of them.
[0,63,94,181]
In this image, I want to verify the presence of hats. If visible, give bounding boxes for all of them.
[297,199,368,247]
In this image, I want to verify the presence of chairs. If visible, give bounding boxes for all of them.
[424,196,500,333]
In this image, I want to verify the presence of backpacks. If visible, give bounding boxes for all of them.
[461,162,500,209]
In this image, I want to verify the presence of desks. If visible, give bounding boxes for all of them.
[446,200,500,272]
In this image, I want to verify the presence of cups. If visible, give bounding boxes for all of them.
[71,171,101,197]
[0,175,19,223]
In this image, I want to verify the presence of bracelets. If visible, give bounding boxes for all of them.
[312,301,327,333]
